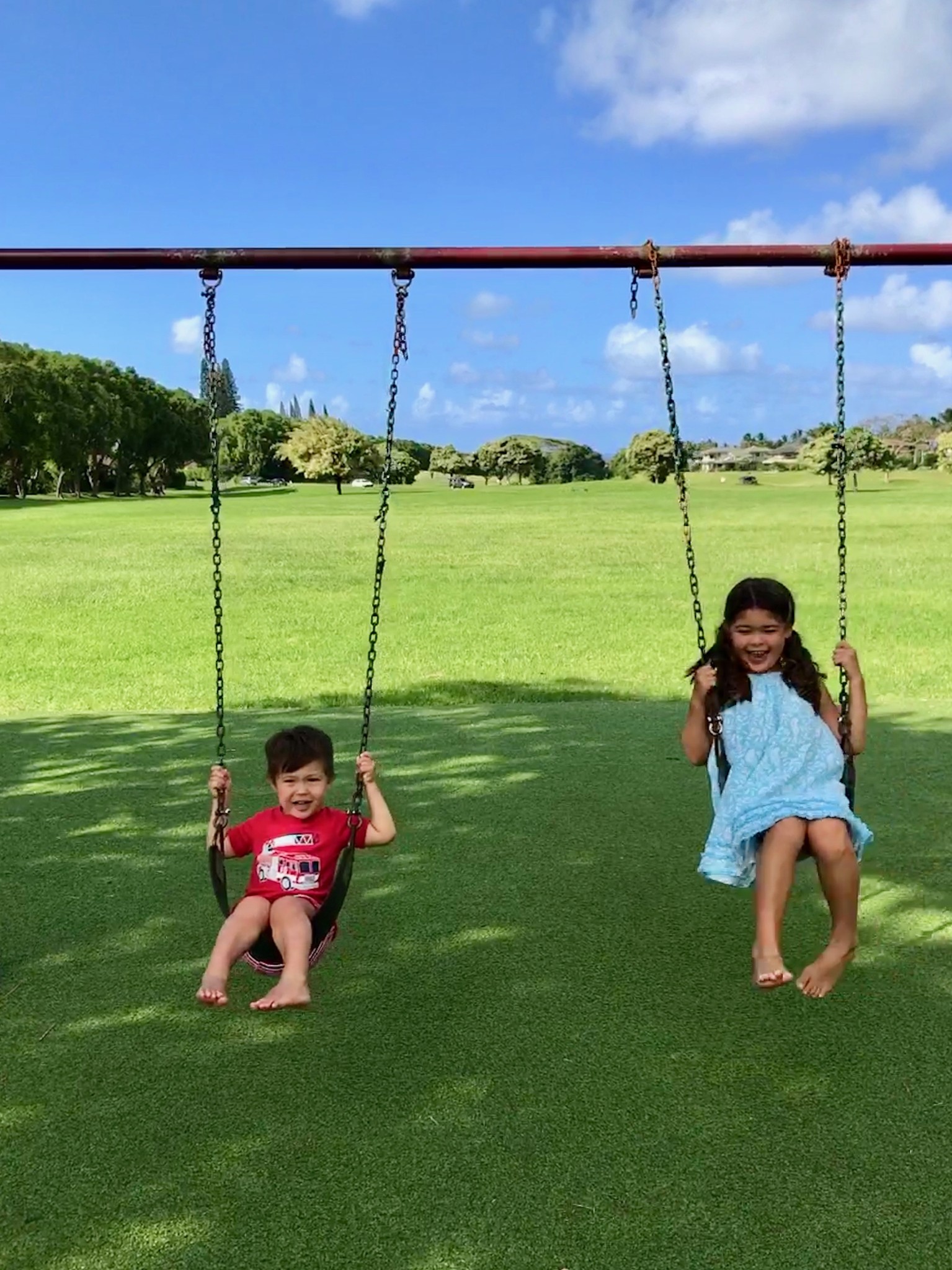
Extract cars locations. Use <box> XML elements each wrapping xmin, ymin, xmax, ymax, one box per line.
<box><xmin>351</xmin><ymin>478</ymin><xmax>374</xmax><ymax>488</ymax></box>
<box><xmin>270</xmin><ymin>478</ymin><xmax>287</xmax><ymax>488</ymax></box>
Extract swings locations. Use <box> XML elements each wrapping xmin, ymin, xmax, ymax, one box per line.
<box><xmin>198</xmin><ymin>272</ymin><xmax>419</xmax><ymax>974</ymax></box>
<box><xmin>628</xmin><ymin>235</ymin><xmax>856</xmax><ymax>814</ymax></box>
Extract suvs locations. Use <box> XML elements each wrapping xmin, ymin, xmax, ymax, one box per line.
<box><xmin>239</xmin><ymin>476</ymin><xmax>257</xmax><ymax>486</ymax></box>
<box><xmin>448</xmin><ymin>476</ymin><xmax>474</xmax><ymax>489</ymax></box>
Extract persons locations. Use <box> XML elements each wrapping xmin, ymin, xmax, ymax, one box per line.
<box><xmin>683</xmin><ymin>578</ymin><xmax>868</xmax><ymax>999</ymax></box>
<box><xmin>197</xmin><ymin>726</ymin><xmax>396</xmax><ymax>1010</ymax></box>
